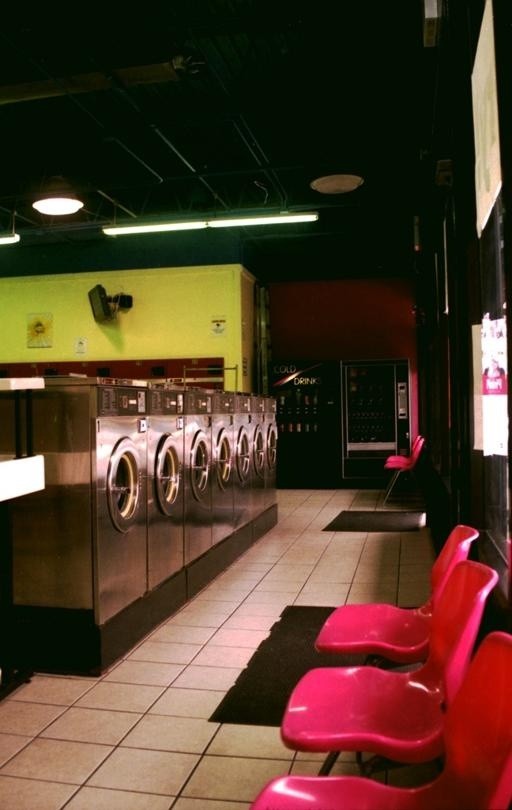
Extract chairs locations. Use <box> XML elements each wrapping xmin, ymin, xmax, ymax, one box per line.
<box><xmin>308</xmin><ymin>524</ymin><xmax>482</xmax><ymax>668</ymax></box>
<box><xmin>276</xmin><ymin>559</ymin><xmax>500</xmax><ymax>775</ymax></box>
<box><xmin>249</xmin><ymin>627</ymin><xmax>512</xmax><ymax>810</ymax></box>
<box><xmin>381</xmin><ymin>435</ymin><xmax>425</xmax><ymax>506</ymax></box>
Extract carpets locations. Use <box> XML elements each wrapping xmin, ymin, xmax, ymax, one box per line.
<box><xmin>208</xmin><ymin>606</ymin><xmax>421</xmax><ymax>726</ymax></box>
<box><xmin>323</xmin><ymin>512</ymin><xmax>426</xmax><ymax>531</ymax></box>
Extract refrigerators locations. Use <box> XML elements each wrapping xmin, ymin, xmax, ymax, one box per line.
<box><xmin>270</xmin><ymin>356</ymin><xmax>412</xmax><ymax>489</ymax></box>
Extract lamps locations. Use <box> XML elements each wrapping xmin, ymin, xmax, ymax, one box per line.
<box><xmin>310</xmin><ymin>173</ymin><xmax>364</xmax><ymax>195</ymax></box>
<box><xmin>31</xmin><ymin>170</ymin><xmax>83</xmax><ymax>221</ymax></box>
<box><xmin>0</xmin><ymin>205</ymin><xmax>21</xmax><ymax>245</ymax></box>
<box><xmin>102</xmin><ymin>199</ymin><xmax>320</xmax><ymax>235</ymax></box>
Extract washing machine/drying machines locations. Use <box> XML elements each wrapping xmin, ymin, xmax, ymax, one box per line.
<box><xmin>0</xmin><ymin>378</ymin><xmax>277</xmax><ymax>678</ymax></box>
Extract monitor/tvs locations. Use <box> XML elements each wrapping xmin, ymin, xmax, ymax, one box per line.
<box><xmin>87</xmin><ymin>285</ymin><xmax>112</xmax><ymax>325</ymax></box>
